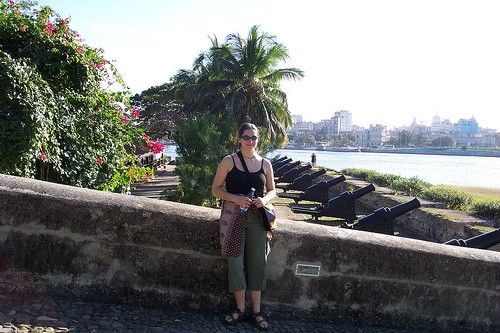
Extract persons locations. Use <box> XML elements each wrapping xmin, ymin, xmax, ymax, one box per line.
<box><xmin>212</xmin><ymin>122</ymin><xmax>277</xmax><ymax>329</ymax></box>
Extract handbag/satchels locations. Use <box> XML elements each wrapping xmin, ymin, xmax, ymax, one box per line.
<box><xmin>260</xmin><ymin>207</ymin><xmax>270</xmax><ymax>231</ymax></box>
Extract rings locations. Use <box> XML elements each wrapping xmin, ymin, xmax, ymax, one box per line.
<box><xmin>258</xmin><ymin>202</ymin><xmax>260</xmax><ymax>204</ymax></box>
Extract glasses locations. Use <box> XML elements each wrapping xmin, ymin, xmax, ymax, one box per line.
<box><xmin>240</xmin><ymin>135</ymin><xmax>258</xmax><ymax>141</ymax></box>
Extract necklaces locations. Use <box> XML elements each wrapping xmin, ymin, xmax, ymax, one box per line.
<box><xmin>243</xmin><ymin>154</ymin><xmax>257</xmax><ymax>162</ymax></box>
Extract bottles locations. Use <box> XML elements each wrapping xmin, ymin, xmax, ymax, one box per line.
<box><xmin>240</xmin><ymin>188</ymin><xmax>255</xmax><ymax>215</ymax></box>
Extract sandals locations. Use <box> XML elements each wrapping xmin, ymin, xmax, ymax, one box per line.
<box><xmin>223</xmin><ymin>307</ymin><xmax>247</xmax><ymax>325</ymax></box>
<box><xmin>250</xmin><ymin>311</ymin><xmax>269</xmax><ymax>330</ymax></box>
